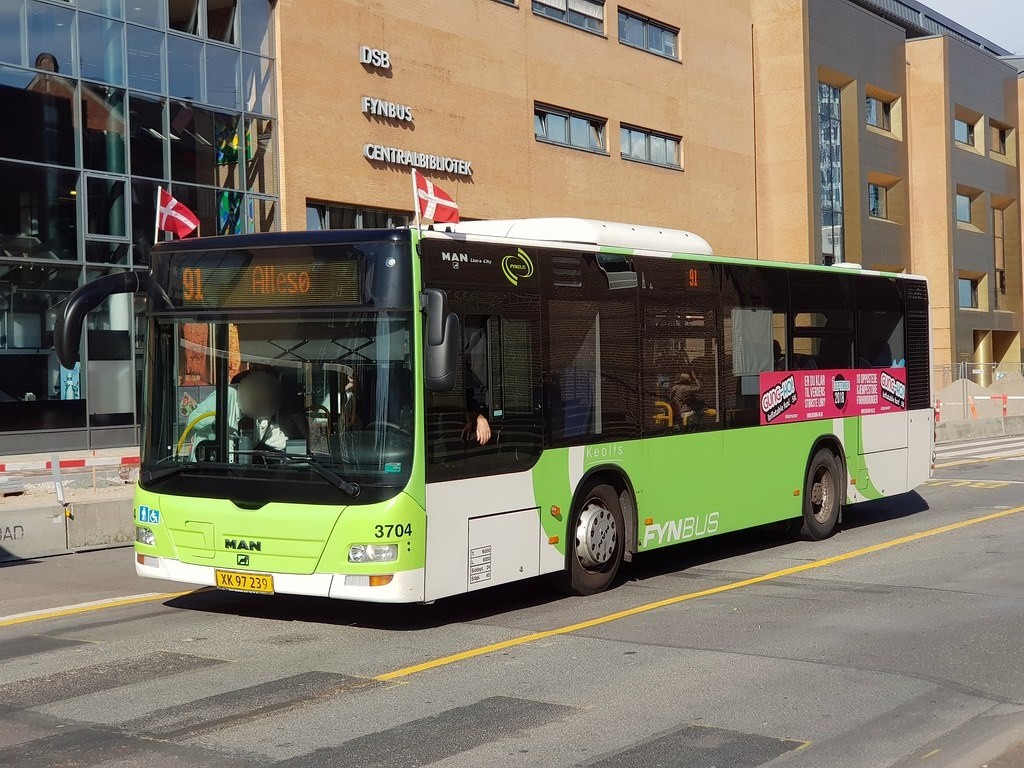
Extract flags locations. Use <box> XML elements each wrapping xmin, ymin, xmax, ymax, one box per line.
<box><xmin>156</xmin><ymin>186</ymin><xmax>200</xmax><ymax>238</ymax></box>
<box><xmin>412</xmin><ymin>169</ymin><xmax>460</xmax><ymax>225</ymax></box>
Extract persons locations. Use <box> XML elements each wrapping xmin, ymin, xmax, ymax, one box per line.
<box><xmin>253</xmin><ymin>410</ymin><xmax>292</xmax><ymax>451</ymax></box>
<box><xmin>669</xmin><ymin>367</ymin><xmax>706</xmax><ymax>432</ymax></box>
<box><xmin>431</xmin><ymin>377</ymin><xmax>491</xmax><ymax>445</ymax></box>
<box><xmin>772</xmin><ymin>339</ymin><xmax>788</xmax><ymax>372</ymax></box>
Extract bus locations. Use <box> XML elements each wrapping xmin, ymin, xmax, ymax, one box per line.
<box><xmin>55</xmin><ymin>216</ymin><xmax>938</xmax><ymax>607</ymax></box>
